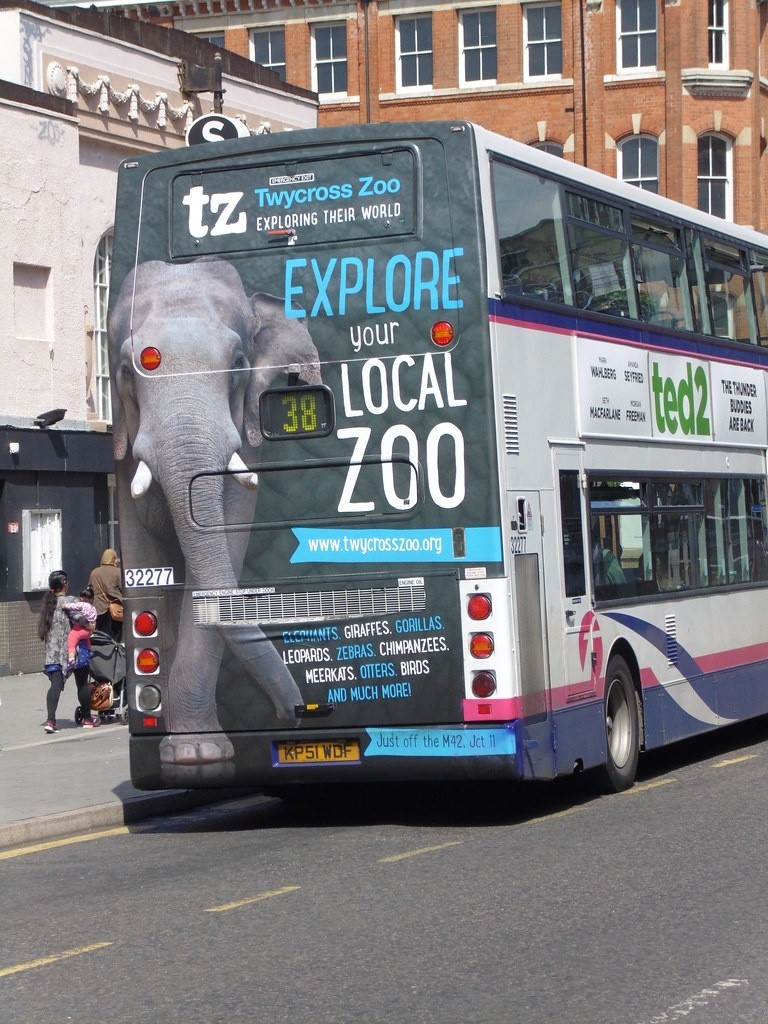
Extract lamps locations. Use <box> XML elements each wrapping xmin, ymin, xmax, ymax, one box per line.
<box><xmin>9</xmin><ymin>442</ymin><xmax>19</xmax><ymax>454</ymax></box>
<box><xmin>33</xmin><ymin>408</ymin><xmax>68</xmax><ymax>427</ymax></box>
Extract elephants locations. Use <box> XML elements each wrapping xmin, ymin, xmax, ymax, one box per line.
<box><xmin>110</xmin><ymin>251</ymin><xmax>322</xmax><ymax>762</ymax></box>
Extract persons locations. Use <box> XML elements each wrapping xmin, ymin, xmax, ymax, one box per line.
<box><xmin>37</xmin><ymin>570</ymin><xmax>101</xmax><ymax>733</ymax></box>
<box><xmin>590</xmin><ymin>533</ymin><xmax>628</xmax><ymax>586</ymax></box>
<box><xmin>89</xmin><ymin>549</ymin><xmax>124</xmax><ymax>642</ymax></box>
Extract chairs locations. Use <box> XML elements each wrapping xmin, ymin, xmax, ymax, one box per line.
<box><xmin>502</xmin><ymin>273</ymin><xmax>678</xmax><ymax>327</ymax></box>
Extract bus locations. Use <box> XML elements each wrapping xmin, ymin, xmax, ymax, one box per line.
<box><xmin>109</xmin><ymin>117</ymin><xmax>768</xmax><ymax>788</ymax></box>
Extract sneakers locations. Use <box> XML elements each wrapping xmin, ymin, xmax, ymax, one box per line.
<box><xmin>44</xmin><ymin>720</ymin><xmax>60</xmax><ymax>732</ymax></box>
<box><xmin>81</xmin><ymin>717</ymin><xmax>101</xmax><ymax>729</ymax></box>
<box><xmin>67</xmin><ymin>659</ymin><xmax>77</xmax><ymax>670</ymax></box>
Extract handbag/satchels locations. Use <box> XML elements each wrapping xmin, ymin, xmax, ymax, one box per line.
<box><xmin>88</xmin><ymin>680</ymin><xmax>114</xmax><ymax>711</ymax></box>
<box><xmin>108</xmin><ymin>602</ymin><xmax>123</xmax><ymax>622</ymax></box>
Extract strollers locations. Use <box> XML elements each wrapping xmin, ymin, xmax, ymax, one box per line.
<box><xmin>75</xmin><ymin>630</ymin><xmax>129</xmax><ymax>725</ymax></box>
